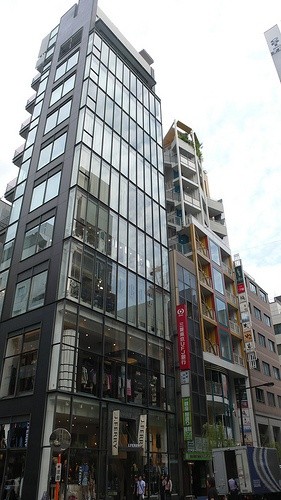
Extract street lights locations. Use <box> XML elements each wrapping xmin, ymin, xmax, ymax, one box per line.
<box><xmin>238</xmin><ymin>382</ymin><xmax>275</xmax><ymax>447</ymax></box>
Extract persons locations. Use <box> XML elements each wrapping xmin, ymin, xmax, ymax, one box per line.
<box><xmin>160</xmin><ymin>476</ymin><xmax>166</xmax><ymax>500</ymax></box>
<box><xmin>137</xmin><ymin>476</ymin><xmax>145</xmax><ymax>500</ymax></box>
<box><xmin>78</xmin><ymin>463</ymin><xmax>89</xmax><ymax>500</ymax></box>
<box><xmin>80</xmin><ymin>364</ymin><xmax>88</xmax><ymax>392</ymax></box>
<box><xmin>228</xmin><ymin>475</ymin><xmax>238</xmax><ymax>500</ymax></box>
<box><xmin>151</xmin><ymin>461</ymin><xmax>160</xmax><ymax>494</ymax></box>
<box><xmin>208</xmin><ymin>478</ymin><xmax>217</xmax><ymax>500</ymax></box>
<box><xmin>130</xmin><ymin>464</ymin><xmax>139</xmax><ymax>495</ymax></box>
<box><xmin>51</xmin><ymin>459</ymin><xmax>64</xmax><ymax>484</ymax></box>
<box><xmin>127</xmin><ymin>377</ymin><xmax>132</xmax><ymax>403</ymax></box>
<box><xmin>165</xmin><ymin>476</ymin><xmax>173</xmax><ymax>500</ymax></box>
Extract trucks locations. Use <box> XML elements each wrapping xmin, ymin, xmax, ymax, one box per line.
<box><xmin>211</xmin><ymin>446</ymin><xmax>281</xmax><ymax>500</ymax></box>
